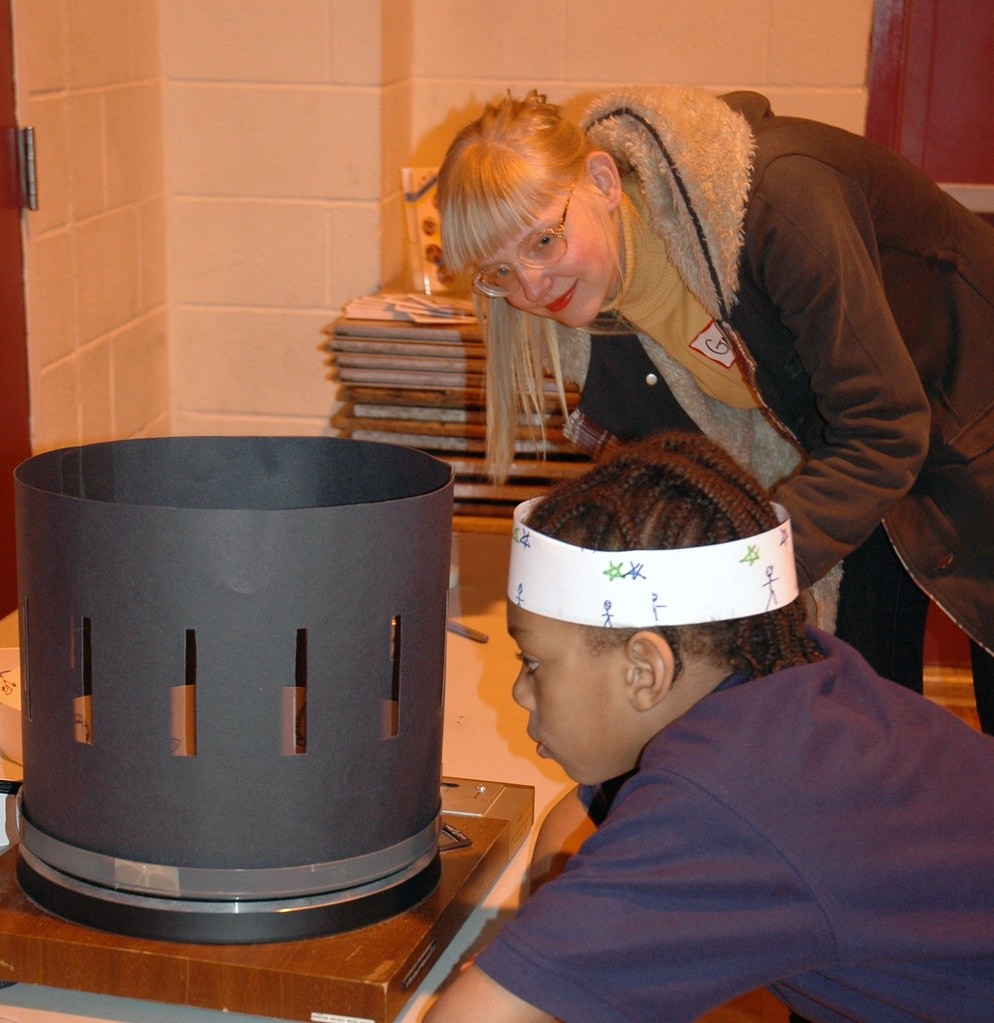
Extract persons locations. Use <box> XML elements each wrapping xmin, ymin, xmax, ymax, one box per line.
<box><xmin>438</xmin><ymin>87</ymin><xmax>994</xmax><ymax>735</ymax></box>
<box><xmin>421</xmin><ymin>434</ymin><xmax>994</xmax><ymax>1023</ymax></box>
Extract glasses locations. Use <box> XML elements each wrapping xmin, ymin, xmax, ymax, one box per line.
<box><xmin>472</xmin><ymin>180</ymin><xmax>574</xmax><ymax>299</ymax></box>
